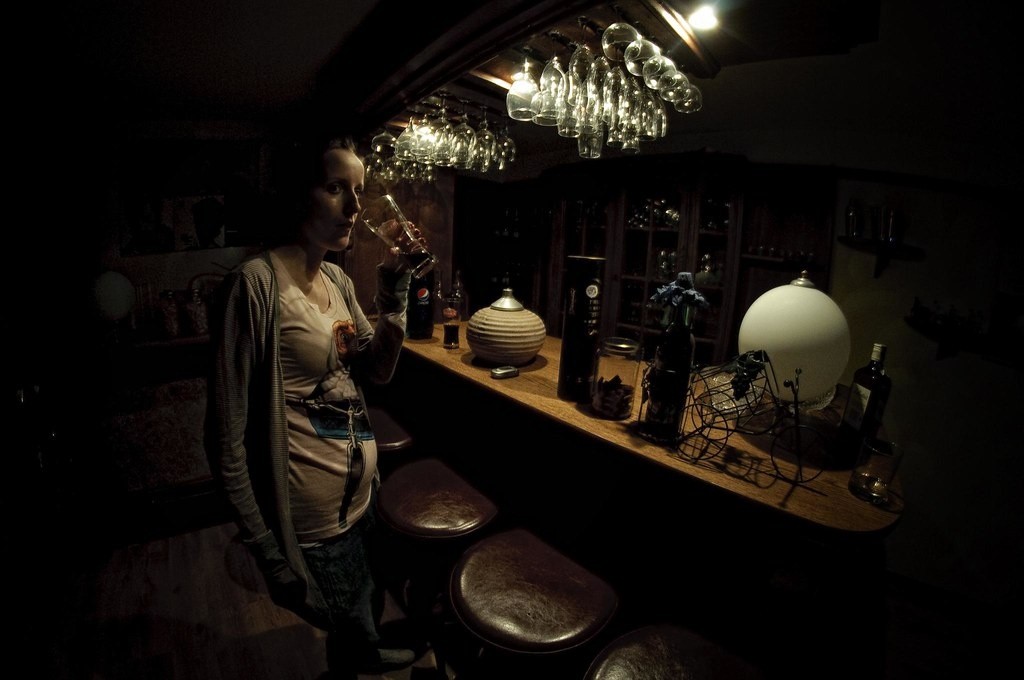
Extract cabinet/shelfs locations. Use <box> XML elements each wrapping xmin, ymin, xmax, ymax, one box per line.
<box><xmin>97</xmin><ymin>138</ymin><xmax>264</xmax><ymax>539</ymax></box>
<box><xmin>460</xmin><ymin>147</ymin><xmax>845</xmax><ymax>368</ymax></box>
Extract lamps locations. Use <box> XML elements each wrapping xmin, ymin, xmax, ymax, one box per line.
<box><xmin>738</xmin><ymin>270</ymin><xmax>850</xmax><ymax>436</ymax></box>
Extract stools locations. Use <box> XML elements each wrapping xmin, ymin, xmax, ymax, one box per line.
<box><xmin>583</xmin><ymin>622</ymin><xmax>763</xmax><ymax>680</ymax></box>
<box><xmin>375</xmin><ymin>457</ymin><xmax>501</xmax><ymax>627</ymax></box>
<box><xmin>450</xmin><ymin>526</ymin><xmax>621</xmax><ymax>657</ymax></box>
<box><xmin>366</xmin><ymin>405</ymin><xmax>415</xmax><ymax>455</ymax></box>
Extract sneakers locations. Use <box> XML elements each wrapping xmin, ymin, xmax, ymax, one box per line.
<box><xmin>376</xmin><ymin>647</ymin><xmax>416</xmax><ymax>665</ymax></box>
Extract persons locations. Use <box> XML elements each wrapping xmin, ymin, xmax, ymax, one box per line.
<box><xmin>201</xmin><ymin>138</ymin><xmax>428</xmax><ymax>680</ymax></box>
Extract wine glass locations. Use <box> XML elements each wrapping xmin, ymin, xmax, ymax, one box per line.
<box><xmin>363</xmin><ymin>86</ymin><xmax>517</xmax><ymax>186</ymax></box>
<box><xmin>500</xmin><ymin>0</ymin><xmax>703</xmax><ymax>160</ymax></box>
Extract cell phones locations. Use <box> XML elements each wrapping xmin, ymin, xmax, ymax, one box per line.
<box><xmin>491</xmin><ymin>366</ymin><xmax>519</xmax><ymax>379</ymax></box>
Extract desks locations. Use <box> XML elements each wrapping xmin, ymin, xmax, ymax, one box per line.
<box><xmin>368</xmin><ymin>319</ymin><xmax>905</xmax><ymax>533</ymax></box>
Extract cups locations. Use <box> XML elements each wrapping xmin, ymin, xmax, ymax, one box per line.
<box><xmin>843</xmin><ymin>198</ymin><xmax>866</xmax><ymax>238</ymax></box>
<box><xmin>359</xmin><ymin>192</ymin><xmax>439</xmax><ymax>280</ymax></box>
<box><xmin>881</xmin><ymin>205</ymin><xmax>906</xmax><ymax>241</ymax></box>
<box><xmin>441</xmin><ymin>297</ymin><xmax>462</xmax><ymax>350</ymax></box>
<box><xmin>847</xmin><ymin>435</ymin><xmax>903</xmax><ymax>506</ymax></box>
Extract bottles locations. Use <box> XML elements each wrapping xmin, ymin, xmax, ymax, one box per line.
<box><xmin>189</xmin><ymin>288</ymin><xmax>210</xmax><ymax>336</ymax></box>
<box><xmin>635</xmin><ymin>269</ymin><xmax>695</xmax><ymax>445</ymax></box>
<box><xmin>403</xmin><ymin>251</ymin><xmax>435</xmax><ymax>340</ymax></box>
<box><xmin>588</xmin><ymin>336</ymin><xmax>639</xmax><ymax>422</ymax></box>
<box><xmin>159</xmin><ymin>289</ymin><xmax>179</xmax><ymax>338</ymax></box>
<box><xmin>838</xmin><ymin>342</ymin><xmax>892</xmax><ymax>445</ymax></box>
